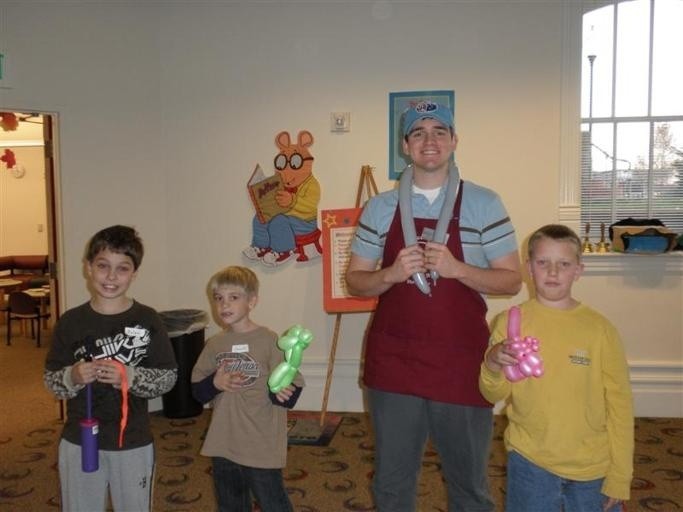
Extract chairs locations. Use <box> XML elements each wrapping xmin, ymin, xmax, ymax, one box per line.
<box><xmin>0</xmin><ymin>255</ymin><xmax>49</xmax><ymax>348</ymax></box>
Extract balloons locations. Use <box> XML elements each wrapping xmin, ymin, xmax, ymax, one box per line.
<box><xmin>399</xmin><ymin>161</ymin><xmax>460</xmax><ymax>296</ymax></box>
<box><xmin>267</xmin><ymin>324</ymin><xmax>314</xmax><ymax>394</ymax></box>
<box><xmin>500</xmin><ymin>306</ymin><xmax>545</xmax><ymax>383</ymax></box>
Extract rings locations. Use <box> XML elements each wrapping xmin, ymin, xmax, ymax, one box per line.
<box><xmin>427</xmin><ymin>256</ymin><xmax>431</xmax><ymax>264</ymax></box>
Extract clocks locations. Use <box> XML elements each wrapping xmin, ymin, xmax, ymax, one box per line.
<box><xmin>12</xmin><ymin>163</ymin><xmax>26</xmax><ymax>178</ymax></box>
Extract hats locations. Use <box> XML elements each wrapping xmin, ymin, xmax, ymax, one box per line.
<box><xmin>403</xmin><ymin>101</ymin><xmax>453</xmax><ymax>136</ymax></box>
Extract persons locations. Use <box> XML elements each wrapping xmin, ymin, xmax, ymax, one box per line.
<box><xmin>478</xmin><ymin>224</ymin><xmax>635</xmax><ymax>511</ymax></box>
<box><xmin>43</xmin><ymin>225</ymin><xmax>178</xmax><ymax>511</ymax></box>
<box><xmin>188</xmin><ymin>265</ymin><xmax>304</xmax><ymax>511</ymax></box>
<box><xmin>343</xmin><ymin>101</ymin><xmax>521</xmax><ymax>511</ymax></box>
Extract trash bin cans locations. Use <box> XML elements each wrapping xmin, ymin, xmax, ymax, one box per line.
<box><xmin>160</xmin><ymin>309</ymin><xmax>206</xmax><ymax>419</ymax></box>
